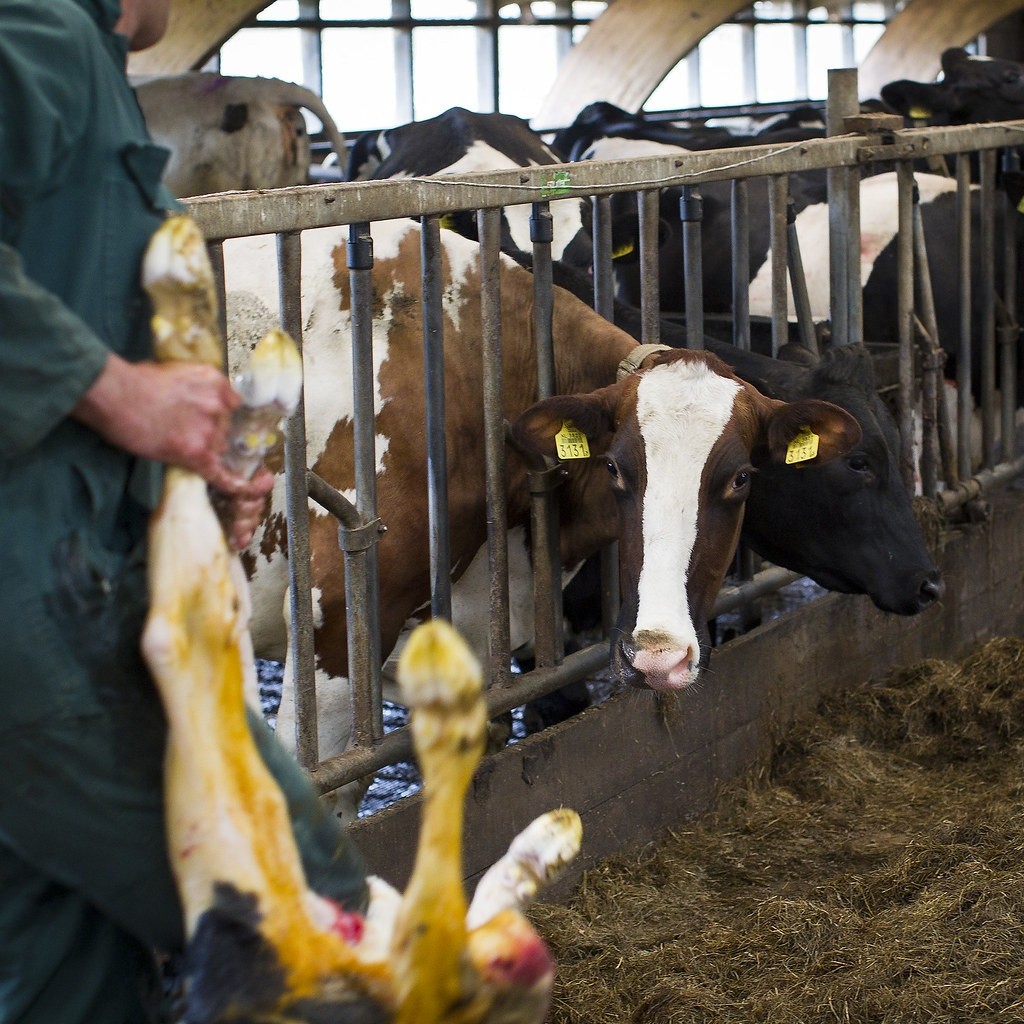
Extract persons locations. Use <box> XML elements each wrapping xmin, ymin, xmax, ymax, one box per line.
<box><xmin>0</xmin><ymin>0</ymin><xmax>373</xmax><ymax>1024</ymax></box>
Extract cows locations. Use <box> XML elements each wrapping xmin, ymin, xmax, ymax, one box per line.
<box><xmin>118</xmin><ymin>48</ymin><xmax>1024</xmax><ymax>820</ymax></box>
